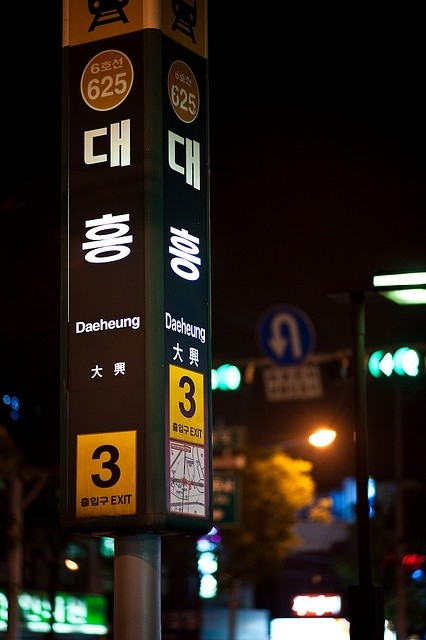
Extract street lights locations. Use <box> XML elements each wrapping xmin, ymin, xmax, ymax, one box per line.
<box><xmin>350</xmin><ymin>260</ymin><xmax>425</xmax><ymax>640</ymax></box>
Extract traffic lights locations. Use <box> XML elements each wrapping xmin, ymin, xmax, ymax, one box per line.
<box><xmin>365</xmin><ymin>346</ymin><xmax>420</xmax><ymax>381</ymax></box>
<box><xmin>210</xmin><ymin>363</ymin><xmax>247</xmax><ymax>392</ymax></box>
